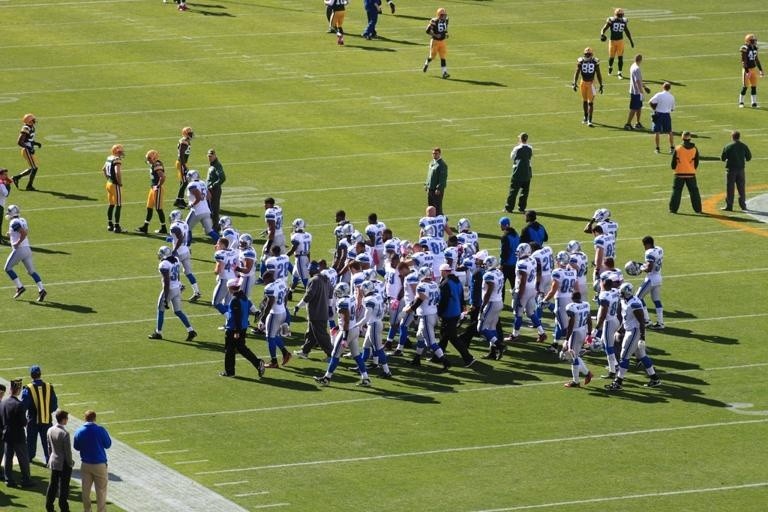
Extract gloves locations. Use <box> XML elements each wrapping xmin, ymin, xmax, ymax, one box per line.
<box><xmin>599</xmin><ymin>86</ymin><xmax>603</xmax><ymax>94</ymax></box>
<box><xmin>746</xmin><ymin>71</ymin><xmax>752</xmax><ymax>79</ymax></box>
<box><xmin>759</xmin><ymin>71</ymin><xmax>764</xmax><ymax>78</ymax></box>
<box><xmin>27</xmin><ymin>146</ymin><xmax>34</xmax><ymax>154</ymax></box>
<box><xmin>639</xmin><ymin>93</ymin><xmax>645</xmax><ymax>101</ymax></box>
<box><xmin>572</xmin><ymin>83</ymin><xmax>578</xmax><ymax>92</ymax></box>
<box><xmin>34</xmin><ymin>142</ymin><xmax>41</xmax><ymax>148</ymax></box>
<box><xmin>644</xmin><ymin>87</ymin><xmax>650</xmax><ymax>94</ymax></box>
<box><xmin>601</xmin><ymin>35</ymin><xmax>607</xmax><ymax>41</ymax></box>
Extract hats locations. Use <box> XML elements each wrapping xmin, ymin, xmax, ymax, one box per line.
<box><xmin>32</xmin><ymin>366</ymin><xmax>40</xmax><ymax>377</ymax></box>
<box><xmin>208</xmin><ymin>149</ymin><xmax>215</xmax><ymax>155</ymax></box>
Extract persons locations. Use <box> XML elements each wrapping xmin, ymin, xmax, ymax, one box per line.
<box><xmin>102</xmin><ymin>128</ymin><xmax>226</xmax><ymax>245</ymax></box>
<box><xmin>323</xmin><ymin>0</ymin><xmax>396</xmax><ymax>45</ymax></box>
<box><xmin>10</xmin><ymin>113</ymin><xmax>41</xmax><ymax>192</ymax></box>
<box><xmin>424</xmin><ymin>148</ymin><xmax>448</xmax><ymax>215</ymax></box>
<box><xmin>572</xmin><ymin>47</ymin><xmax>605</xmax><ymax>128</ymax></box>
<box><xmin>669</xmin><ymin>131</ymin><xmax>702</xmax><ymax>214</ymax></box>
<box><xmin>422</xmin><ymin>8</ymin><xmax>451</xmax><ymax>79</ymax></box>
<box><xmin>500</xmin><ymin>133</ymin><xmax>533</xmax><ymax>212</ymax></box>
<box><xmin>1</xmin><ymin>366</ymin><xmax>111</xmax><ymax>512</ymax></box>
<box><xmin>0</xmin><ymin>204</ymin><xmax>47</xmax><ymax>302</ymax></box>
<box><xmin>649</xmin><ymin>81</ymin><xmax>676</xmax><ymax>155</ymax></box>
<box><xmin>0</xmin><ymin>169</ymin><xmax>13</xmax><ymax>244</ymax></box>
<box><xmin>174</xmin><ymin>0</ymin><xmax>188</xmax><ymax>12</ymax></box>
<box><xmin>624</xmin><ymin>55</ymin><xmax>650</xmax><ymax>131</ymax></box>
<box><xmin>600</xmin><ymin>8</ymin><xmax>634</xmax><ymax>80</ymax></box>
<box><xmin>721</xmin><ymin>132</ymin><xmax>752</xmax><ymax>212</ymax></box>
<box><xmin>148</xmin><ymin>170</ymin><xmax>665</xmax><ymax>391</ymax></box>
<box><xmin>738</xmin><ymin>34</ymin><xmax>765</xmax><ymax>109</ymax></box>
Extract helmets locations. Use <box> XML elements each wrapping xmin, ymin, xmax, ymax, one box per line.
<box><xmin>590</xmin><ymin>336</ymin><xmax>604</xmax><ymax>351</ymax></box>
<box><xmin>681</xmin><ymin>131</ymin><xmax>691</xmax><ymax>140</ymax></box>
<box><xmin>158</xmin><ymin>206</ymin><xmax>582</xmax><ymax>299</ymax></box>
<box><xmin>744</xmin><ymin>34</ymin><xmax>757</xmax><ymax>47</ymax></box>
<box><xmin>620</xmin><ymin>282</ymin><xmax>635</xmax><ymax>299</ymax></box>
<box><xmin>625</xmin><ymin>261</ymin><xmax>642</xmax><ymax>276</ymax></box>
<box><xmin>615</xmin><ymin>8</ymin><xmax>625</xmax><ymax>19</ymax></box>
<box><xmin>436</xmin><ymin>7</ymin><xmax>447</xmax><ymax>21</ymax></box>
<box><xmin>595</xmin><ymin>209</ymin><xmax>611</xmax><ymax>221</ymax></box>
<box><xmin>23</xmin><ymin>114</ymin><xmax>38</xmax><ymax>129</ymax></box>
<box><xmin>6</xmin><ymin>204</ymin><xmax>19</xmax><ymax>218</ymax></box>
<box><xmin>111</xmin><ymin>126</ymin><xmax>200</xmax><ymax>182</ymax></box>
<box><xmin>582</xmin><ymin>47</ymin><xmax>595</xmax><ymax>60</ymax></box>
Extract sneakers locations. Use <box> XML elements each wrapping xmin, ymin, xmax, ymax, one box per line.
<box><xmin>647</xmin><ymin>320</ymin><xmax>652</xmax><ymax>330</ymax></box>
<box><xmin>1</xmin><ymin>239</ymin><xmax>8</xmax><ymax>244</ymax></box>
<box><xmin>36</xmin><ymin>289</ymin><xmax>47</xmax><ymax>301</ymax></box>
<box><xmin>544</xmin><ymin>344</ymin><xmax>560</xmax><ymax>354</ymax></box>
<box><xmin>669</xmin><ymin>207</ymin><xmax>746</xmax><ymax>214</ymax></box>
<box><xmin>584</xmin><ymin>370</ymin><xmax>593</xmax><ymax>385</ymax></box>
<box><xmin>649</xmin><ymin>322</ymin><xmax>665</xmax><ymax>329</ymax></box>
<box><xmin>26</xmin><ymin>185</ymin><xmax>35</xmax><ymax>191</ymax></box>
<box><xmin>106</xmin><ymin>198</ymin><xmax>188</xmax><ymax>234</ymax></box>
<box><xmin>423</xmin><ymin>65</ymin><xmax>428</xmax><ymax>73</ymax></box>
<box><xmin>185</xmin><ymin>331</ymin><xmax>197</xmax><ymax>342</ymax></box>
<box><xmin>564</xmin><ymin>381</ymin><xmax>580</xmax><ymax>388</ymax></box>
<box><xmin>552</xmin><ymin>306</ymin><xmax>556</xmax><ymax>320</ymax></box>
<box><xmin>189</xmin><ymin>292</ymin><xmax>202</xmax><ymax>303</ymax></box>
<box><xmin>11</xmin><ymin>176</ymin><xmax>18</xmax><ymax>188</ymax></box>
<box><xmin>443</xmin><ymin>72</ymin><xmax>450</xmax><ymax>79</ymax></box>
<box><xmin>739</xmin><ymin>104</ymin><xmax>744</xmax><ymax>108</ymax></box>
<box><xmin>326</xmin><ymin>26</ymin><xmax>380</xmax><ymax>45</ymax></box>
<box><xmin>217</xmin><ymin>300</ymin><xmax>547</xmax><ymax>387</ymax></box>
<box><xmin>179</xmin><ymin>5</ymin><xmax>186</xmax><ymax>11</ymax></box>
<box><xmin>599</xmin><ymin>357</ymin><xmax>662</xmax><ymax>391</ymax></box>
<box><xmin>149</xmin><ymin>330</ymin><xmax>162</xmax><ymax>341</ymax></box>
<box><xmin>13</xmin><ymin>287</ymin><xmax>25</xmax><ymax>298</ymax></box>
<box><xmin>582</xmin><ymin>67</ymin><xmax>675</xmax><ymax>154</ymax></box>
<box><xmin>752</xmin><ymin>105</ymin><xmax>760</xmax><ymax>109</ymax></box>
